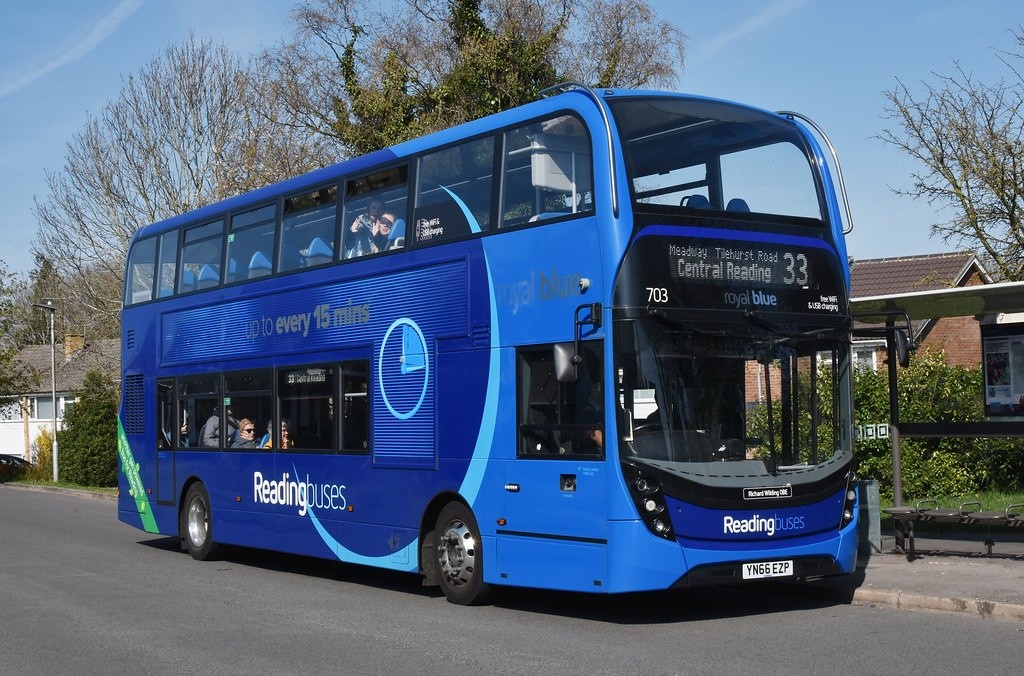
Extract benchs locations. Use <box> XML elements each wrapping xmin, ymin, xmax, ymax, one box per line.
<box><xmin>882</xmin><ymin>500</ymin><xmax>1024</xmax><ymax>563</ymax></box>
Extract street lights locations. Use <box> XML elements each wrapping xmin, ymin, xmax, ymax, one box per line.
<box><xmin>33</xmin><ymin>303</ymin><xmax>58</xmax><ymax>483</ymax></box>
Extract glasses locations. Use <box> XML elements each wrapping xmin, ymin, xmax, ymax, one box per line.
<box><xmin>243</xmin><ymin>428</ymin><xmax>256</xmax><ymax>433</ymax></box>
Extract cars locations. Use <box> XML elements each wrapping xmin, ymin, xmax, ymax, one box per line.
<box><xmin>0</xmin><ymin>453</ymin><xmax>35</xmax><ymax>483</ymax></box>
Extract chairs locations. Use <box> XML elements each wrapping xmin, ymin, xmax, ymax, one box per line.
<box><xmin>681</xmin><ymin>195</ymin><xmax>712</xmax><ymax>208</ymax></box>
<box><xmin>726</xmin><ymin>197</ymin><xmax>750</xmax><ymax>212</ymax></box>
<box><xmin>184</xmin><ymin>429</ymin><xmax>270</xmax><ymax>448</ymax></box>
<box><xmin>158</xmin><ymin>220</ymin><xmax>407</xmax><ymax>298</ymax></box>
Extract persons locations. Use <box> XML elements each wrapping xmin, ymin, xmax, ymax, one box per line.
<box><xmin>163</xmin><ymin>397</ymin><xmax>296</xmax><ymax>454</ymax></box>
<box><xmin>992</xmin><ymin>367</ymin><xmax>1008</xmax><ymax>385</ymax></box>
<box><xmin>344</xmin><ymin>196</ymin><xmax>398</xmax><ymax>259</ymax></box>
<box><xmin>572</xmin><ymin>379</ymin><xmax>623</xmax><ymax>455</ymax></box>
<box><xmin>645</xmin><ymin>386</ymin><xmax>687</xmax><ymax>450</ymax></box>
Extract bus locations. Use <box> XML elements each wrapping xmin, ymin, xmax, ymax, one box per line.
<box><xmin>117</xmin><ymin>80</ymin><xmax>915</xmax><ymax>607</ymax></box>
